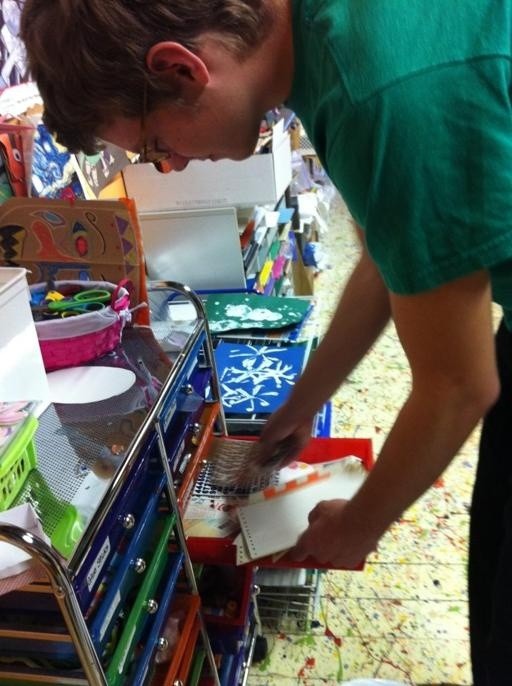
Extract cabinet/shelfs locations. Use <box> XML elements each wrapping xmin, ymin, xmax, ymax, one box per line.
<box><xmin>0</xmin><ymin>279</ymin><xmax>268</xmax><ymax>686</ymax></box>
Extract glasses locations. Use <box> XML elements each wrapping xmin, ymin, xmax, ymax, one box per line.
<box><xmin>132</xmin><ymin>79</ymin><xmax>171</xmax><ymax>164</ymax></box>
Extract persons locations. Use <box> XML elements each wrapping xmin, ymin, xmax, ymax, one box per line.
<box><xmin>17</xmin><ymin>1</ymin><xmax>511</xmax><ymax>680</ymax></box>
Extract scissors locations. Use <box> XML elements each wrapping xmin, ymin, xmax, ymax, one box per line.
<box><xmin>32</xmin><ymin>289</ymin><xmax>111</xmax><ymax>316</ymax></box>
